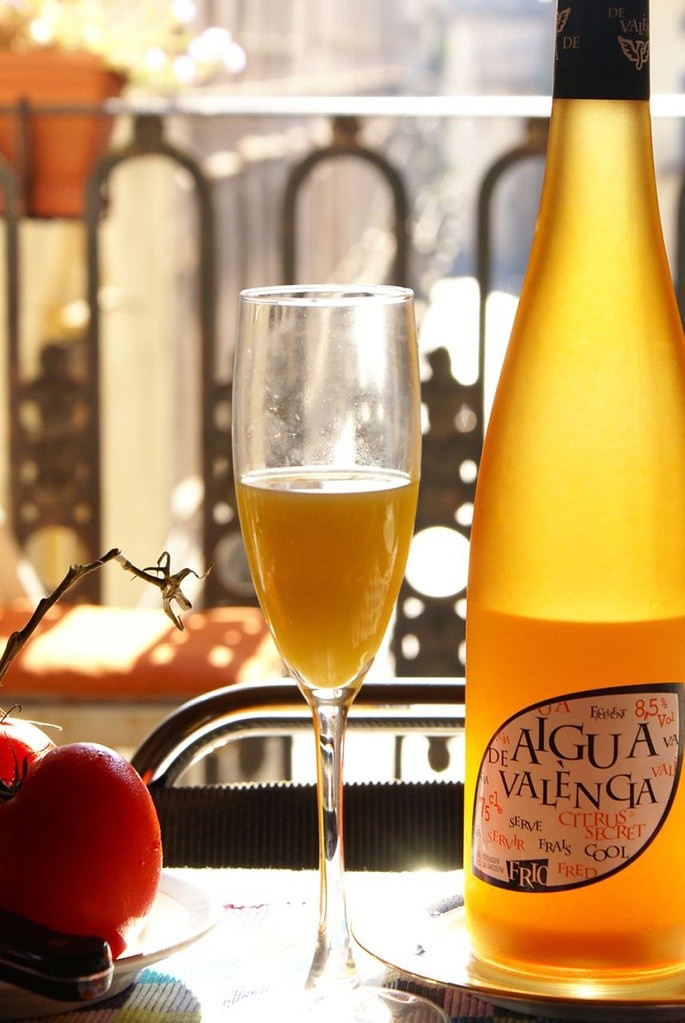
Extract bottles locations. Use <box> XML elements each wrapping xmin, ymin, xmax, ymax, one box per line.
<box><xmin>457</xmin><ymin>0</ymin><xmax>685</xmax><ymax>995</ymax></box>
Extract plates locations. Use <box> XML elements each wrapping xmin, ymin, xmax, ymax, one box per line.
<box><xmin>0</xmin><ymin>874</ymin><xmax>221</xmax><ymax>1012</ymax></box>
<box><xmin>344</xmin><ymin>863</ymin><xmax>684</xmax><ymax>1020</ymax></box>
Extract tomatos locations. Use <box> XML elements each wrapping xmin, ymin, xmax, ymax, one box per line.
<box><xmin>0</xmin><ymin>705</ymin><xmax>164</xmax><ymax>957</ymax></box>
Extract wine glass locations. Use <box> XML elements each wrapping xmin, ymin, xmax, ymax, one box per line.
<box><xmin>222</xmin><ymin>277</ymin><xmax>448</xmax><ymax>1023</ymax></box>
